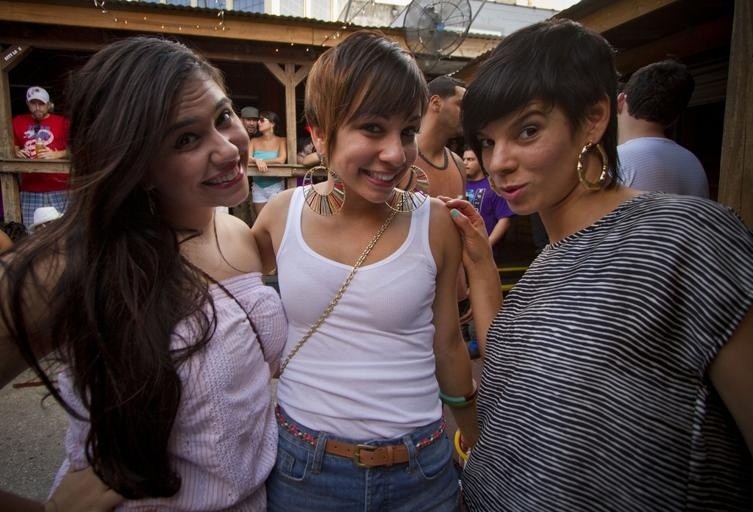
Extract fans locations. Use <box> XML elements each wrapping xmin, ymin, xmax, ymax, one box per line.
<box><xmin>401</xmin><ymin>1</ymin><xmax>472</xmax><ymax>61</ymax></box>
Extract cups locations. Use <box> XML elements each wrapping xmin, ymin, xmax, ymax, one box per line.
<box><xmin>34</xmin><ymin>137</ymin><xmax>45</xmax><ymax>159</ymax></box>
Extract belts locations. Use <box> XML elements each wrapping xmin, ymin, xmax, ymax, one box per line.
<box><xmin>321</xmin><ymin>439</ymin><xmax>408</xmax><ymax>467</ymax></box>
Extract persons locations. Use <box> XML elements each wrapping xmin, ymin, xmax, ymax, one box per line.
<box><xmin>297</xmin><ymin>136</ymin><xmax>319</xmax><ymax>187</ymax></box>
<box><xmin>230</xmin><ymin>104</ymin><xmax>258</xmax><ymax>229</ymax></box>
<box><xmin>0</xmin><ymin>32</ymin><xmax>288</xmax><ymax>511</ymax></box>
<box><xmin>610</xmin><ymin>60</ymin><xmax>711</xmax><ymax>200</ymax></box>
<box><xmin>396</xmin><ymin>74</ymin><xmax>472</xmax><ymax>327</ymax></box>
<box><xmin>11</xmin><ymin>83</ymin><xmax>78</xmax><ymax>236</ymax></box>
<box><xmin>435</xmin><ymin>17</ymin><xmax>753</xmax><ymax>512</ymax></box>
<box><xmin>26</xmin><ymin>204</ymin><xmax>65</xmax><ymax>239</ymax></box>
<box><xmin>248</xmin><ymin>110</ymin><xmax>288</xmax><ymax>277</ymax></box>
<box><xmin>247</xmin><ymin>29</ymin><xmax>484</xmax><ymax>512</ymax></box>
<box><xmin>460</xmin><ymin>146</ymin><xmax>513</xmax><ymax>361</ymax></box>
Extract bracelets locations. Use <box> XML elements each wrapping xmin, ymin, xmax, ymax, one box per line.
<box><xmin>437</xmin><ymin>378</ymin><xmax>479</xmax><ymax>408</ymax></box>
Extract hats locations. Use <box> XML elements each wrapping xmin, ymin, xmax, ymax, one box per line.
<box><xmin>241</xmin><ymin>107</ymin><xmax>259</xmax><ymax>118</ymax></box>
<box><xmin>27</xmin><ymin>87</ymin><xmax>49</xmax><ymax>104</ymax></box>
<box><xmin>28</xmin><ymin>207</ymin><xmax>63</xmax><ymax>231</ymax></box>
